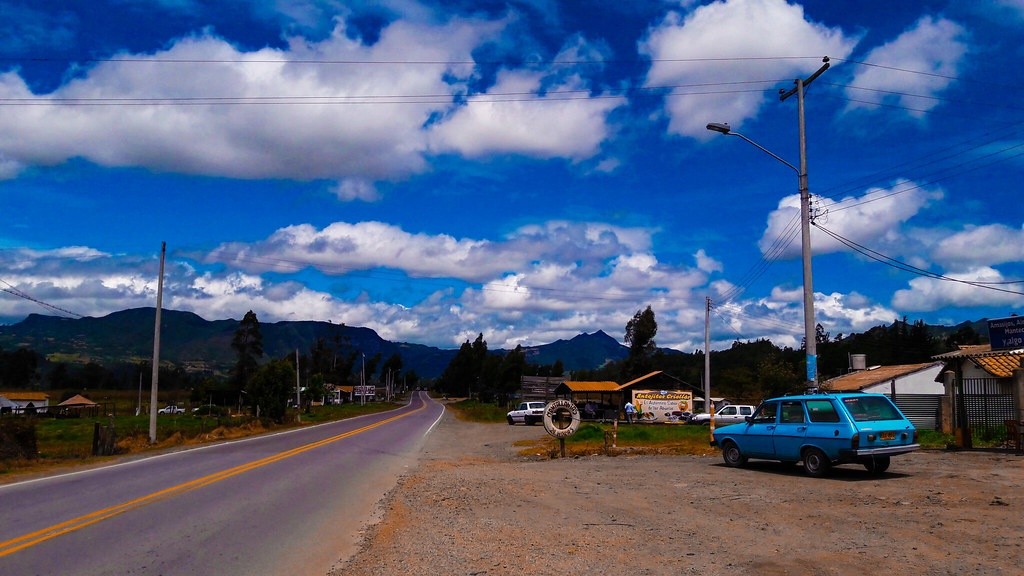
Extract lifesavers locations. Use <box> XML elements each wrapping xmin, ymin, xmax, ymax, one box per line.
<box><xmin>542</xmin><ymin>399</ymin><xmax>581</xmax><ymax>438</ymax></box>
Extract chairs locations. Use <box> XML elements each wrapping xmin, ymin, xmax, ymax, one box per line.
<box><xmin>1005</xmin><ymin>419</ymin><xmax>1024</xmax><ymax>456</ymax></box>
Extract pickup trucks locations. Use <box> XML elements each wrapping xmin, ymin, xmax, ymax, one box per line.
<box><xmin>157</xmin><ymin>405</ymin><xmax>186</xmax><ymax>414</ymax></box>
<box><xmin>691</xmin><ymin>404</ymin><xmax>781</xmax><ymax>430</ymax></box>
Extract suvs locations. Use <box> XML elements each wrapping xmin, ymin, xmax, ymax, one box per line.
<box><xmin>192</xmin><ymin>403</ymin><xmax>218</xmax><ymax>416</ymax></box>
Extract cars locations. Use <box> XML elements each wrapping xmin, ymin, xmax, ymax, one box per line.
<box><xmin>506</xmin><ymin>401</ymin><xmax>557</xmax><ymax>426</ymax></box>
<box><xmin>710</xmin><ymin>388</ymin><xmax>921</xmax><ymax>477</ymax></box>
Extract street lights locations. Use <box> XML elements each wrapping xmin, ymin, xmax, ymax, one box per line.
<box><xmin>707</xmin><ymin>54</ymin><xmax>839</xmax><ymax>393</ymax></box>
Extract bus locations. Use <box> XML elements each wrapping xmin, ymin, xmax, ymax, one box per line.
<box><xmin>328</xmin><ymin>388</ymin><xmax>349</xmax><ymax>406</ymax></box>
<box><xmin>309</xmin><ymin>392</ymin><xmax>327</xmax><ymax>406</ymax></box>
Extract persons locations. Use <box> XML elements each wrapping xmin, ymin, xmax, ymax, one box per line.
<box><xmin>583</xmin><ymin>399</ymin><xmax>612</xmax><ymax>420</ymax></box>
<box><xmin>624</xmin><ymin>400</ymin><xmax>636</xmax><ymax>424</ymax></box>
